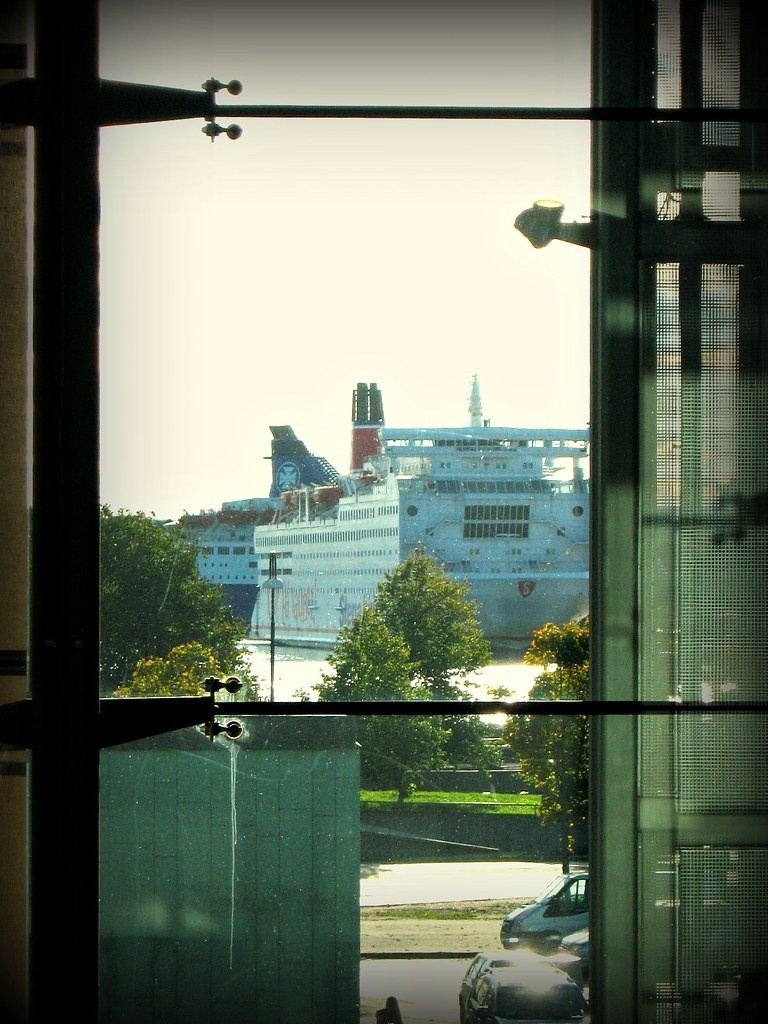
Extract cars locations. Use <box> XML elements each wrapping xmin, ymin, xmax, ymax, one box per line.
<box><xmin>458</xmin><ymin>949</ymin><xmax>591</xmax><ymax>1024</ymax></box>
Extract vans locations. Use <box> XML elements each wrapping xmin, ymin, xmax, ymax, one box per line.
<box><xmin>558</xmin><ymin>897</ymin><xmax>726</xmax><ymax>968</ymax></box>
<box><xmin>497</xmin><ymin>870</ymin><xmax>676</xmax><ymax>949</ymax></box>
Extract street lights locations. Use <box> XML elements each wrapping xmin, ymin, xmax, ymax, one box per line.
<box><xmin>262</xmin><ymin>552</ymin><xmax>286</xmax><ymax>702</ymax></box>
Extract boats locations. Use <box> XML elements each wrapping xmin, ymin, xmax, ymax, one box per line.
<box><xmin>312</xmin><ymin>485</ymin><xmax>342</xmax><ymax>506</ymax></box>
<box><xmin>278</xmin><ymin>490</ymin><xmax>293</xmax><ymax>505</ymax></box>
<box><xmin>292</xmin><ymin>486</ymin><xmax>314</xmax><ymax>504</ymax></box>
<box><xmin>236</xmin><ymin>638</ymin><xmax>278</xmax><ymax>648</ymax></box>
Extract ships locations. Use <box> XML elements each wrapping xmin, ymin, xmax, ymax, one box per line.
<box><xmin>250</xmin><ymin>373</ymin><xmax>592</xmax><ymax>650</ymax></box>
